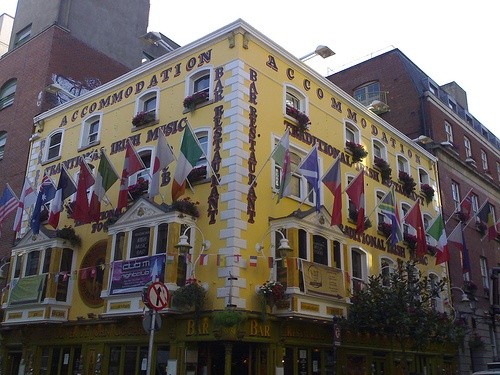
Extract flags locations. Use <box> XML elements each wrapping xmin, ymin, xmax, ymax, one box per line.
<box><xmin>117</xmin><ymin>141</ymin><xmax>146</xmax><ymax>214</ymax></box>
<box><xmin>272</xmin><ymin>132</ymin><xmax>291</xmax><ymax>204</ymax></box>
<box><xmin>28</xmin><ymin>173</ymin><xmax>57</xmax><ymax>233</ymax></box>
<box><xmin>381</xmin><ymin>186</ymin><xmax>403</xmax><ymax>247</ymax></box>
<box><xmin>301</xmin><ymin>147</ymin><xmax>322</xmax><ymax>213</ymax></box>
<box><xmin>449</xmin><ymin>224</ymin><xmax>472</xmax><ymax>273</ymax></box>
<box><xmin>324</xmin><ymin>157</ymin><xmax>344</xmax><ymax>227</ymax></box>
<box><xmin>346</xmin><ymin>170</ymin><xmax>366</xmax><ymax>235</ymax></box>
<box><xmin>172</xmin><ymin>124</ymin><xmax>204</xmax><ymax>200</ymax></box>
<box><xmin>167</xmin><ymin>252</ymin><xmax>301</xmax><ymax>269</ymax></box>
<box><xmin>88</xmin><ymin>153</ymin><xmax>119</xmax><ymax>223</ymax></box>
<box><xmin>54</xmin><ymin>264</ymin><xmax>105</xmax><ymax>283</ymax></box>
<box><xmin>427</xmin><ymin>213</ymin><xmax>451</xmax><ymax>265</ymax></box>
<box><xmin>48</xmin><ymin>166</ymin><xmax>76</xmax><ymax>229</ymax></box>
<box><xmin>406</xmin><ymin>201</ymin><xmax>428</xmax><ymax>257</ymax></box>
<box><xmin>72</xmin><ymin>160</ymin><xmax>95</xmax><ymax>223</ymax></box>
<box><xmin>149</xmin><ymin>126</ymin><xmax>173</xmax><ymax>202</ymax></box>
<box><xmin>479</xmin><ymin>202</ymin><xmax>498</xmax><ymax>241</ymax></box>
<box><xmin>463</xmin><ymin>191</ymin><xmax>473</xmax><ymax>217</ymax></box>
<box><xmin>0</xmin><ymin>185</ymin><xmax>19</xmax><ymax>225</ymax></box>
<box><xmin>13</xmin><ymin>178</ymin><xmax>36</xmax><ymax>230</ymax></box>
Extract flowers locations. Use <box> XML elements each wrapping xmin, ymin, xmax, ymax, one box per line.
<box><xmin>403</xmin><ymin>233</ymin><xmax>416</xmax><ymax>245</ymax></box>
<box><xmin>398</xmin><ymin>170</ymin><xmax>416</xmax><ymax>190</ymax></box>
<box><xmin>376</xmin><ymin>222</ymin><xmax>393</xmax><ymax>236</ymax></box>
<box><xmin>103</xmin><ymin>166</ymin><xmax>208</xmax><ymax>232</ymax></box>
<box><xmin>346</xmin><ymin>140</ymin><xmax>368</xmax><ymax>163</ymax></box>
<box><xmin>286</xmin><ymin>106</ymin><xmax>311</xmax><ymax>132</ymax></box>
<box><xmin>132</xmin><ymin>91</ymin><xmax>208</xmax><ymax>127</ymax></box>
<box><xmin>255</xmin><ymin>279</ymin><xmax>287</xmax><ymax>311</ymax></box>
<box><xmin>421</xmin><ymin>184</ymin><xmax>489</xmax><ymax>233</ymax></box>
<box><xmin>374</xmin><ymin>155</ymin><xmax>392</xmax><ymax>181</ymax></box>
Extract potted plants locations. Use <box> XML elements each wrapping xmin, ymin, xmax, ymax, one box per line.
<box><xmin>56</xmin><ymin>225</ymin><xmax>82</xmax><ymax>248</ymax></box>
<box><xmin>174</xmin><ymin>279</ymin><xmax>206</xmax><ymax>313</ymax></box>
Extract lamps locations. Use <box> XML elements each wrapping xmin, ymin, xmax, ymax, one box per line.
<box><xmin>368</xmin><ymin>100</ymin><xmax>390</xmax><ymax>113</ymax></box>
<box><xmin>466</xmin><ymin>158</ymin><xmax>477</xmax><ymax>164</ymax></box>
<box><xmin>255</xmin><ymin>229</ymin><xmax>292</xmax><ymax>253</ymax></box>
<box><xmin>413</xmin><ymin>135</ymin><xmax>433</xmax><ymax>143</ymax></box>
<box><xmin>299</xmin><ymin>45</ymin><xmax>335</xmax><ymax>62</ymax></box>
<box><xmin>441</xmin><ymin>142</ymin><xmax>453</xmax><ymax>147</ymax></box>
<box><xmin>447</xmin><ymin>287</ymin><xmax>469</xmax><ymax>301</ymax></box>
<box><xmin>173</xmin><ymin>226</ymin><xmax>209</xmax><ymax>251</ymax></box>
